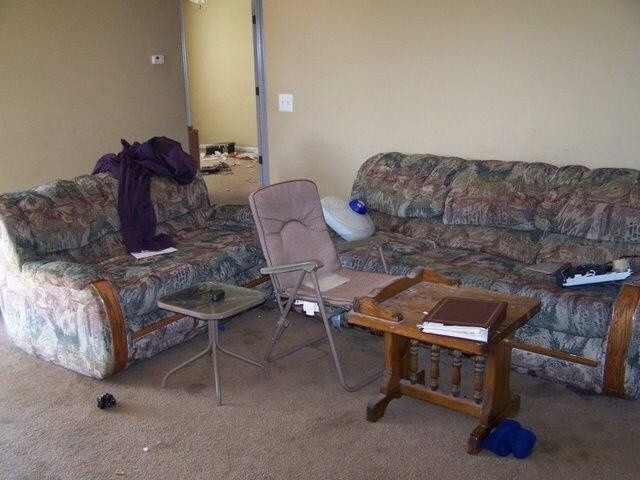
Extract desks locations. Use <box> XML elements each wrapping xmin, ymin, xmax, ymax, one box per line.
<box><xmin>157</xmin><ymin>281</ymin><xmax>266</xmax><ymax>406</ymax></box>
<box><xmin>346</xmin><ymin>265</ymin><xmax>541</xmax><ymax>455</ymax></box>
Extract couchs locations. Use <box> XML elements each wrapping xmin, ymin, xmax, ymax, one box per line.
<box><xmin>296</xmin><ymin>151</ymin><xmax>640</xmax><ymax>400</ymax></box>
<box><xmin>0</xmin><ymin>164</ymin><xmax>280</xmax><ymax>378</ymax></box>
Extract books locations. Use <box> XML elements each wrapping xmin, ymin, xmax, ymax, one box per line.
<box><xmin>417</xmin><ymin>296</ymin><xmax>508</xmax><ymax>343</ymax></box>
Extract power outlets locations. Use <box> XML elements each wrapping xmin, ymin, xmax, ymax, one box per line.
<box><xmin>278</xmin><ymin>94</ymin><xmax>293</xmax><ymax>112</ymax></box>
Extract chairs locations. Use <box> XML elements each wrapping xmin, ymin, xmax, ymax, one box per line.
<box><xmin>248</xmin><ymin>179</ymin><xmax>401</xmax><ymax>393</ymax></box>
